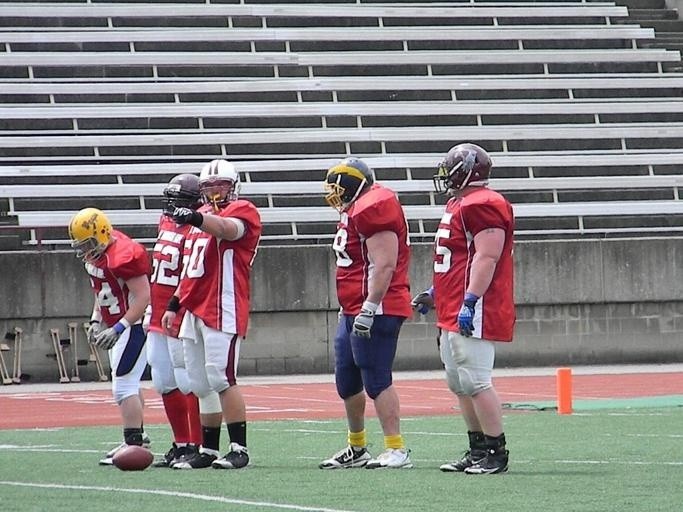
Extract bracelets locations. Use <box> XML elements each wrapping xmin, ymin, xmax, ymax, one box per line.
<box><xmin>166</xmin><ymin>296</ymin><xmax>179</xmax><ymax>313</ymax></box>
<box><xmin>90</xmin><ymin>311</ymin><xmax>103</xmax><ymax>323</ymax></box>
<box><xmin>190</xmin><ymin>211</ymin><xmax>203</xmax><ymax>227</ymax></box>
<box><xmin>362</xmin><ymin>302</ymin><xmax>378</xmax><ymax>313</ymax></box>
<box><xmin>113</xmin><ymin>322</ymin><xmax>126</xmax><ymax>334</ymax></box>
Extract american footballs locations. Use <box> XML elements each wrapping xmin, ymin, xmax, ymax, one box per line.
<box><xmin>112</xmin><ymin>445</ymin><xmax>154</xmax><ymax>471</ymax></box>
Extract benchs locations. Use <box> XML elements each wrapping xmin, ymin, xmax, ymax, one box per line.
<box><xmin>0</xmin><ymin>0</ymin><xmax>683</xmax><ymax>249</ymax></box>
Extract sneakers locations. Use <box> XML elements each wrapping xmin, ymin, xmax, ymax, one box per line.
<box><xmin>318</xmin><ymin>445</ymin><xmax>372</xmax><ymax>469</ymax></box>
<box><xmin>366</xmin><ymin>448</ymin><xmax>413</xmax><ymax>469</ymax></box>
<box><xmin>153</xmin><ymin>442</ymin><xmax>248</xmax><ymax>469</ymax></box>
<box><xmin>440</xmin><ymin>450</ymin><xmax>509</xmax><ymax>473</ymax></box>
<box><xmin>99</xmin><ymin>430</ymin><xmax>152</xmax><ymax>465</ymax></box>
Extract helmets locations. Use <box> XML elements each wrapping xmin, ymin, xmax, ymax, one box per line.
<box><xmin>198</xmin><ymin>160</ymin><xmax>241</xmax><ymax>212</ymax></box>
<box><xmin>325</xmin><ymin>157</ymin><xmax>373</xmax><ymax>214</ymax></box>
<box><xmin>433</xmin><ymin>143</ymin><xmax>492</xmax><ymax>197</ymax></box>
<box><xmin>160</xmin><ymin>174</ymin><xmax>204</xmax><ymax>223</ymax></box>
<box><xmin>68</xmin><ymin>208</ymin><xmax>114</xmax><ymax>264</ymax></box>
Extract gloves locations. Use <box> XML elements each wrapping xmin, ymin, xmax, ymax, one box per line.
<box><xmin>352</xmin><ymin>301</ymin><xmax>377</xmax><ymax>339</ymax></box>
<box><xmin>410</xmin><ymin>288</ymin><xmax>435</xmax><ymax>314</ymax></box>
<box><xmin>164</xmin><ymin>204</ymin><xmax>203</xmax><ymax>227</ymax></box>
<box><xmin>458</xmin><ymin>293</ymin><xmax>478</xmax><ymax>338</ymax></box>
<box><xmin>87</xmin><ymin>322</ymin><xmax>120</xmax><ymax>350</ymax></box>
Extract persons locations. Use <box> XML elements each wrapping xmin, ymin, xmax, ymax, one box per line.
<box><xmin>162</xmin><ymin>159</ymin><xmax>262</xmax><ymax>470</ymax></box>
<box><xmin>68</xmin><ymin>207</ymin><xmax>151</xmax><ymax>465</ymax></box>
<box><xmin>409</xmin><ymin>142</ymin><xmax>516</xmax><ymax>473</ymax></box>
<box><xmin>143</xmin><ymin>173</ymin><xmax>203</xmax><ymax>468</ymax></box>
<box><xmin>319</xmin><ymin>157</ymin><xmax>414</xmax><ymax>470</ymax></box>
